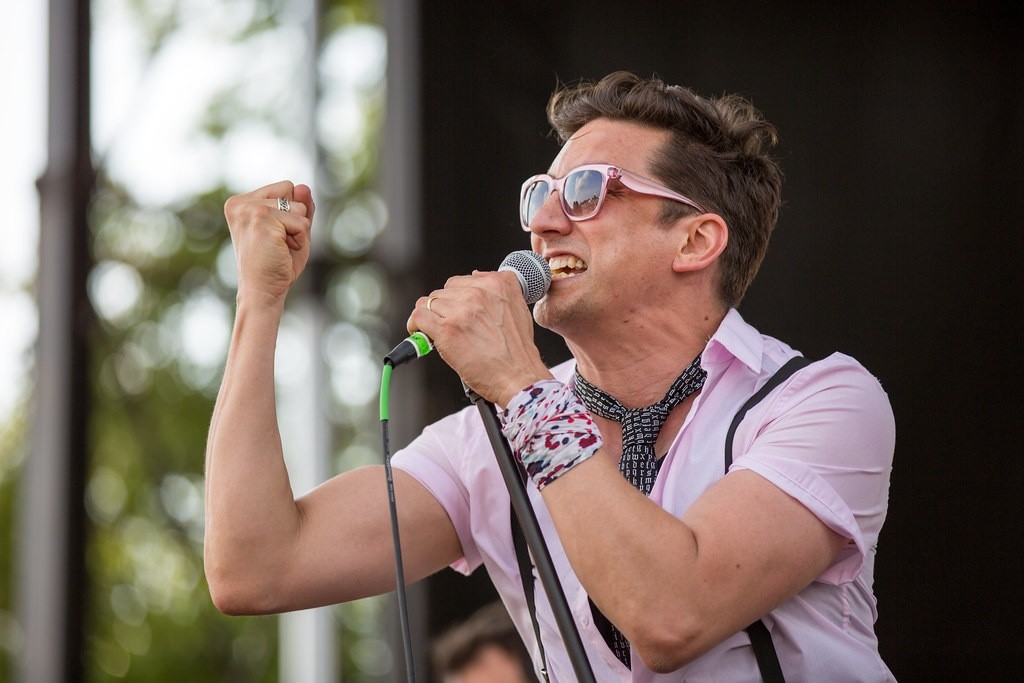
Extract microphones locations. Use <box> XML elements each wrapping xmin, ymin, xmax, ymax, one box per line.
<box><xmin>383</xmin><ymin>250</ymin><xmax>552</xmax><ymax>369</ymax></box>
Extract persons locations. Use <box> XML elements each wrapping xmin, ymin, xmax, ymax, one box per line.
<box><xmin>427</xmin><ymin>599</ymin><xmax>540</xmax><ymax>683</ymax></box>
<box><xmin>201</xmin><ymin>72</ymin><xmax>900</xmax><ymax>683</ymax></box>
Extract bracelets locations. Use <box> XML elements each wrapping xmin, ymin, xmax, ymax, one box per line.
<box><xmin>496</xmin><ymin>381</ymin><xmax>605</xmax><ymax>491</ymax></box>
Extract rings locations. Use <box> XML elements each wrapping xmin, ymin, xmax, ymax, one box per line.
<box><xmin>427</xmin><ymin>296</ymin><xmax>439</xmax><ymax>310</ymax></box>
<box><xmin>277</xmin><ymin>197</ymin><xmax>290</xmax><ymax>211</ymax></box>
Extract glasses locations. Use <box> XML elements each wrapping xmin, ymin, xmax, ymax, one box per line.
<box><xmin>519</xmin><ymin>162</ymin><xmax>709</xmax><ymax>233</ymax></box>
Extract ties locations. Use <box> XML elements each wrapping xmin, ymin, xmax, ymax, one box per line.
<box><xmin>572</xmin><ymin>347</ymin><xmax>708</xmax><ymax>673</ymax></box>
